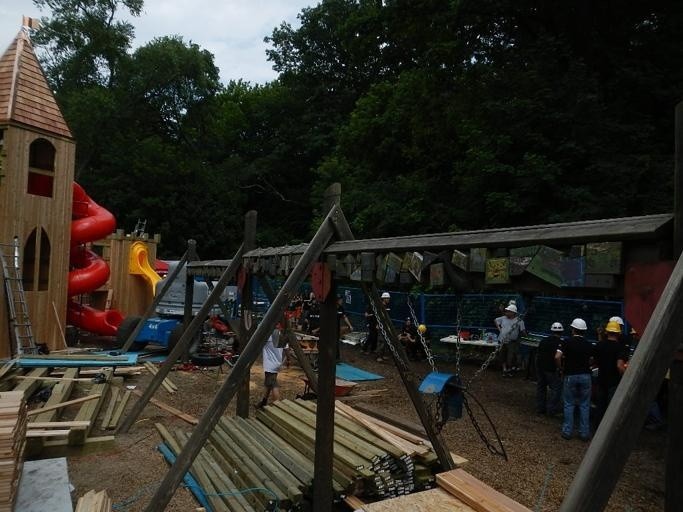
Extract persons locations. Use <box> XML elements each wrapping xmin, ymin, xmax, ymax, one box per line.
<box><xmin>334</xmin><ymin>294</ymin><xmax>353</xmax><ymax>364</ymax></box>
<box><xmin>300</xmin><ymin>297</ymin><xmax>321</xmax><ymax>372</ymax></box>
<box><xmin>252</xmin><ymin>323</ymin><xmax>290</xmax><ymax>409</ymax></box>
<box><xmin>300</xmin><ymin>292</ymin><xmax>316</xmax><ymax>333</ymax></box>
<box><xmin>373</xmin><ymin>290</ymin><xmax>394</xmax><ymax>363</ymax></box>
<box><xmin>406</xmin><ymin>324</ymin><xmax>432</xmax><ymax>360</ymax></box>
<box><xmin>396</xmin><ymin>316</ymin><xmax>419</xmax><ymax>357</ymax></box>
<box><xmin>628</xmin><ymin>328</ymin><xmax>641</xmax><ymax>345</ymax></box>
<box><xmin>553</xmin><ymin>318</ymin><xmax>598</xmax><ymax>441</ymax></box>
<box><xmin>592</xmin><ymin>322</ymin><xmax>628</xmax><ymax>412</ymax></box>
<box><xmin>504</xmin><ymin>282</ymin><xmax>527</xmax><ymax>372</ymax></box>
<box><xmin>358</xmin><ymin>304</ymin><xmax>378</xmax><ymax>355</ymax></box>
<box><xmin>607</xmin><ymin>315</ymin><xmax>630</xmax><ymax>365</ymax></box>
<box><xmin>495</xmin><ymin>299</ymin><xmax>533</xmax><ymax>378</ymax></box>
<box><xmin>532</xmin><ymin>321</ymin><xmax>565</xmax><ymax>418</ymax></box>
<box><xmin>645</xmin><ymin>401</ymin><xmax>663</xmax><ymax>430</ymax></box>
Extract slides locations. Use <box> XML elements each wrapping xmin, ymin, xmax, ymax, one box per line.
<box><xmin>69</xmin><ymin>181</ymin><xmax>126</xmax><ymax>337</ymax></box>
<box><xmin>128</xmin><ymin>241</ymin><xmax>163</xmax><ymax>298</ymax></box>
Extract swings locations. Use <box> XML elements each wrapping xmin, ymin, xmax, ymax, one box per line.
<box><xmin>191</xmin><ymin>288</ymin><xmax>225</xmax><ymax>367</ymax></box>
<box><xmin>366</xmin><ymin>282</ymin><xmax>529</xmax><ymax>460</ymax></box>
<box><xmin>273</xmin><ymin>269</ymin><xmax>302</xmax><ymax>349</ymax></box>
<box><xmin>241</xmin><ymin>264</ymin><xmax>259</xmax><ymax>333</ymax></box>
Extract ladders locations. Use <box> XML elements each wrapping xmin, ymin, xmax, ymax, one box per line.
<box><xmin>0</xmin><ymin>235</ymin><xmax>39</xmax><ymax>356</ymax></box>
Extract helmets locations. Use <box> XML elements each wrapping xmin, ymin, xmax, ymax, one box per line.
<box><xmin>336</xmin><ymin>294</ymin><xmax>342</xmax><ymax>301</ymax></box>
<box><xmin>505</xmin><ymin>304</ymin><xmax>516</xmax><ymax>312</ymax></box>
<box><xmin>605</xmin><ymin>317</ymin><xmax>624</xmax><ymax>333</ymax></box>
<box><xmin>380</xmin><ymin>293</ymin><xmax>391</xmax><ymax>299</ymax></box>
<box><xmin>405</xmin><ymin>316</ymin><xmax>410</xmax><ymax>321</ymax></box>
<box><xmin>570</xmin><ymin>318</ymin><xmax>587</xmax><ymax>330</ymax></box>
<box><xmin>550</xmin><ymin>323</ymin><xmax>563</xmax><ymax>331</ymax></box>
<box><xmin>418</xmin><ymin>325</ymin><xmax>425</xmax><ymax>333</ymax></box>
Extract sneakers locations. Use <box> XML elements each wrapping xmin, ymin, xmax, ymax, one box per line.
<box><xmin>367</xmin><ymin>354</ymin><xmax>388</xmax><ymax>362</ymax></box>
<box><xmin>562</xmin><ymin>433</ymin><xmax>589</xmax><ymax>440</ymax></box>
<box><xmin>538</xmin><ymin>410</ymin><xmax>561</xmax><ymax>417</ymax></box>
<box><xmin>254</xmin><ymin>401</ymin><xmax>263</xmax><ymax>407</ymax></box>
<box><xmin>502</xmin><ymin>366</ymin><xmax>520</xmax><ymax>376</ymax></box>
<box><xmin>645</xmin><ymin>417</ymin><xmax>661</xmax><ymax>431</ymax></box>
